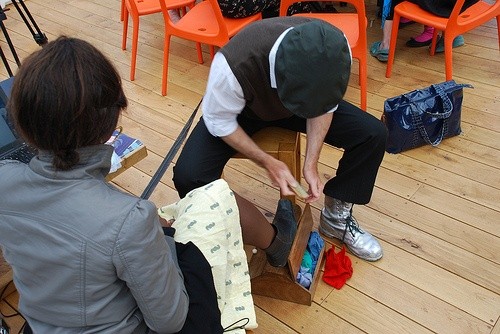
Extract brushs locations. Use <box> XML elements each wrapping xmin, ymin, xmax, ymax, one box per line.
<box><xmin>289</xmin><ymin>183</ymin><xmax>310</xmax><ymax>199</ymax></box>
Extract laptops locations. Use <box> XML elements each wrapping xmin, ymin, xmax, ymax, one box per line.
<box><xmin>0</xmin><ymin>86</ymin><xmax>39</xmax><ymax>164</ymax></box>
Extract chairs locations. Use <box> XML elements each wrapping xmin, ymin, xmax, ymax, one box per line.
<box><xmin>386</xmin><ymin>0</ymin><xmax>500</xmax><ymax>81</ymax></box>
<box><xmin>280</xmin><ymin>0</ymin><xmax>367</xmax><ymax>112</ymax></box>
<box><xmin>121</xmin><ymin>0</ymin><xmax>262</xmax><ymax>96</ymax></box>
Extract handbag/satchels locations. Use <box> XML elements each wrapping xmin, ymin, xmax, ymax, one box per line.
<box><xmin>379</xmin><ymin>79</ymin><xmax>474</xmax><ymax>154</ymax></box>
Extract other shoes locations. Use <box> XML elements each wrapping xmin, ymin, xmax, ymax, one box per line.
<box><xmin>405</xmin><ymin>35</ymin><xmax>439</xmax><ymax>48</ymax></box>
<box><xmin>435</xmin><ymin>34</ymin><xmax>465</xmax><ymax>53</ymax></box>
<box><xmin>370</xmin><ymin>41</ymin><xmax>389</xmax><ymax>62</ymax></box>
<box><xmin>398</xmin><ymin>20</ymin><xmax>415</xmax><ymax>29</ymax></box>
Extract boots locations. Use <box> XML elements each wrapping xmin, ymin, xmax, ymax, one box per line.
<box><xmin>317</xmin><ymin>195</ymin><xmax>384</xmax><ymax>263</ymax></box>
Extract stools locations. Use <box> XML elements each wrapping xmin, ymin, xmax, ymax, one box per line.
<box><xmin>220</xmin><ymin>126</ymin><xmax>300</xmax><ymax>203</ymax></box>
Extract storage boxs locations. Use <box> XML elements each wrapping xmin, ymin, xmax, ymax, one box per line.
<box><xmin>248</xmin><ymin>203</ymin><xmax>327</xmax><ymax>306</ymax></box>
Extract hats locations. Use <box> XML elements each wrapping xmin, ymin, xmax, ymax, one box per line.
<box><xmin>275</xmin><ymin>21</ymin><xmax>352</xmax><ymax>119</ymax></box>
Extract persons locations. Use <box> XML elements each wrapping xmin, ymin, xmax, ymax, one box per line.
<box><xmin>171</xmin><ymin>17</ymin><xmax>390</xmax><ymax>268</ymax></box>
<box><xmin>0</xmin><ymin>34</ymin><xmax>225</xmax><ymax>334</ymax></box>
<box><xmin>370</xmin><ymin>0</ymin><xmax>480</xmax><ymax>63</ymax></box>
<box><xmin>216</xmin><ymin>0</ymin><xmax>338</xmax><ymax>20</ymax></box>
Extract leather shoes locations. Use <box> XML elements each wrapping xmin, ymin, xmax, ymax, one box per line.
<box><xmin>263</xmin><ymin>199</ymin><xmax>297</xmax><ymax>268</ymax></box>
